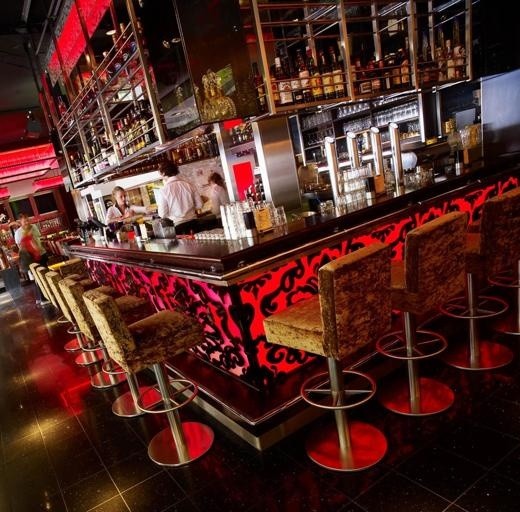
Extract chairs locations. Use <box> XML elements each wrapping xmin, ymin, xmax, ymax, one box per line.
<box><xmin>450</xmin><ymin>188</ymin><xmax>520</xmax><ymax>337</ymax></box>
<box><xmin>45</xmin><ymin>272</ymin><xmax>99</xmax><ymax>364</ymax></box>
<box><xmin>59</xmin><ymin>278</ymin><xmax>148</xmax><ymax>420</ymax></box>
<box><xmin>262</xmin><ymin>242</ymin><xmax>392</xmax><ymax>471</ymax></box>
<box><xmin>82</xmin><ymin>288</ymin><xmax>214</xmax><ymax>469</ymax></box>
<box><xmin>28</xmin><ymin>257</ymin><xmax>79</xmax><ymax>324</ymax></box>
<box><xmin>378</xmin><ymin>210</ymin><xmax>471</xmax><ymax>417</ymax></box>
<box><xmin>37</xmin><ymin>266</ymin><xmax>87</xmax><ymax>350</ymax></box>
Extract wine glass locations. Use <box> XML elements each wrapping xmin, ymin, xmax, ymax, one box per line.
<box><xmin>306</xmin><ymin>127</ymin><xmax>334</xmax><ymax>145</ymax></box>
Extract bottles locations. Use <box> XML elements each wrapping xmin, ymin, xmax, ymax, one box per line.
<box><xmin>167</xmin><ymin>136</ymin><xmax>216</xmax><ymax>163</ymax></box>
<box><xmin>101</xmin><ymin>19</ymin><xmax>138</xmax><ymax>84</ymax></box>
<box><xmin>69</xmin><ymin>151</ymin><xmax>91</xmax><ymax>184</ymax></box>
<box><xmin>87</xmin><ymin>136</ymin><xmax>119</xmax><ymax>175</ymax></box>
<box><xmin>71</xmin><ymin>71</ymin><xmax>98</xmax><ymax>115</ymax></box>
<box><xmin>269</xmin><ymin>51</ymin><xmax>345</xmax><ymax>108</ymax></box>
<box><xmin>351</xmin><ymin>50</ymin><xmax>411</xmax><ymax>93</ymax></box>
<box><xmin>114</xmin><ymin>100</ymin><xmax>158</xmax><ymax>158</ymax></box>
<box><xmin>253</xmin><ymin>59</ymin><xmax>268</xmax><ymax>110</ymax></box>
<box><xmin>454</xmin><ymin>145</ymin><xmax>465</xmax><ymax>178</ymax></box>
<box><xmin>136</xmin><ymin>20</ymin><xmax>148</xmax><ymax>50</ymax></box>
<box><xmin>57</xmin><ymin>96</ymin><xmax>71</xmax><ymax>126</ymax></box>
<box><xmin>414</xmin><ymin>39</ymin><xmax>468</xmax><ymax>86</ymax></box>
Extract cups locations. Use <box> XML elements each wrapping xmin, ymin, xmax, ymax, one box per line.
<box><xmin>336</xmin><ymin>103</ymin><xmax>370</xmax><ymax>119</ymax></box>
<box><xmin>301</xmin><ymin>112</ymin><xmax>334</xmax><ymax>127</ymax></box>
<box><xmin>254</xmin><ymin>198</ymin><xmax>287</xmax><ymax>232</ymax></box>
<box><xmin>371</xmin><ymin>102</ymin><xmax>418</xmax><ymax>126</ymax></box>
<box><xmin>340</xmin><ymin>116</ymin><xmax>372</xmax><ymax>134</ymax></box>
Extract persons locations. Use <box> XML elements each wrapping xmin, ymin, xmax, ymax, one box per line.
<box><xmin>298</xmin><ymin>156</ymin><xmax>316</xmax><ymax>190</ymax></box>
<box><xmin>14</xmin><ymin>211</ymin><xmax>48</xmax><ymax>271</ymax></box>
<box><xmin>208</xmin><ymin>172</ymin><xmax>228</xmax><ymax>219</ymax></box>
<box><xmin>20</xmin><ymin>224</ymin><xmax>51</xmax><ymax>306</ymax></box>
<box><xmin>106</xmin><ymin>186</ymin><xmax>146</xmax><ymax>230</ymax></box>
<box><xmin>155</xmin><ymin>161</ymin><xmax>204</xmax><ymax>237</ymax></box>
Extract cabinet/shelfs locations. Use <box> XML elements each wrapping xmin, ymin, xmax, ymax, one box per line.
<box><xmin>370</xmin><ymin>89</ymin><xmax>425</xmax><ymax>143</ymax></box>
<box><xmin>299</xmin><ymin>111</ymin><xmax>340</xmax><ymax>158</ymax></box>
<box><xmin>242</xmin><ymin>0</ymin><xmax>474</xmax><ymax>116</ymax></box>
<box><xmin>86</xmin><ymin>112</ymin><xmax>300</xmax><ymax>222</ymax></box>
<box><xmin>35</xmin><ymin>1</ymin><xmax>203</xmax><ymax>188</ymax></box>
<box><xmin>1</xmin><ymin>209</ymin><xmax>61</xmax><ymax>244</ymax></box>
<box><xmin>330</xmin><ymin>100</ymin><xmax>371</xmax><ymax>153</ymax></box>
